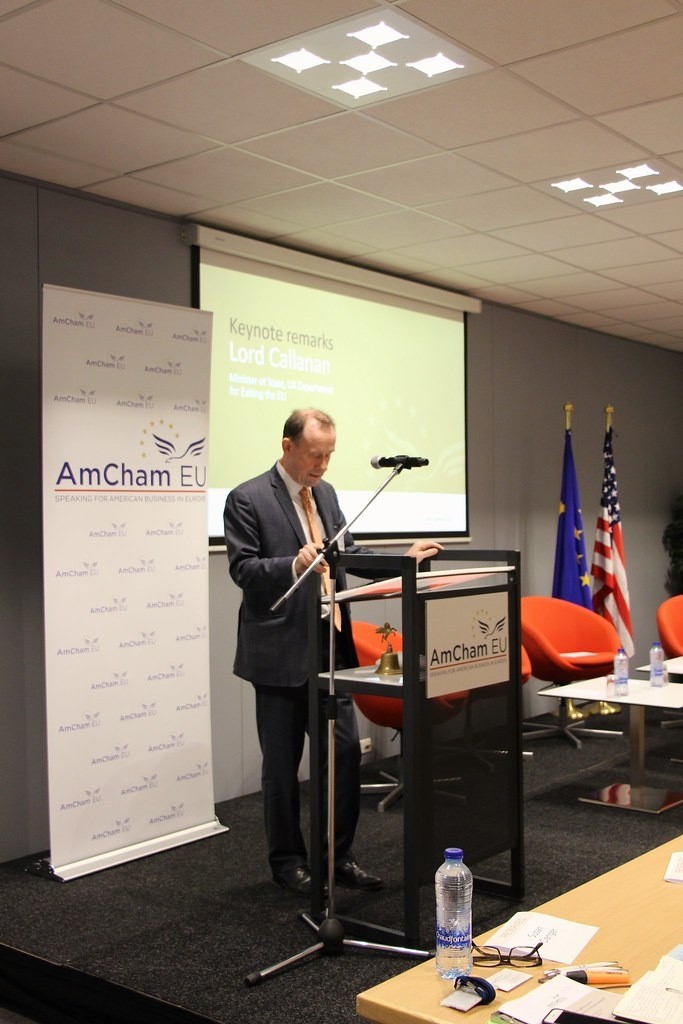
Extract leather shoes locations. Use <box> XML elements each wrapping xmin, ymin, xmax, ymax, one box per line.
<box><xmin>273</xmin><ymin>866</ymin><xmax>328</xmax><ymax>897</ymax></box>
<box><xmin>333</xmin><ymin>861</ymin><xmax>384</xmax><ymax>889</ymax></box>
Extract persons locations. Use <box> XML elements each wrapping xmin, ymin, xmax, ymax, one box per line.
<box><xmin>223</xmin><ymin>408</ymin><xmax>444</xmax><ymax>891</ymax></box>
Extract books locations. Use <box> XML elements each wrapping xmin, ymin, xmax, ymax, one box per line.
<box><xmin>489</xmin><ymin>942</ymin><xmax>683</xmax><ymax>1024</ymax></box>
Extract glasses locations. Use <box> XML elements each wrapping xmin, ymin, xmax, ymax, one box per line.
<box><xmin>470</xmin><ymin>940</ymin><xmax>544</xmax><ymax>967</ymax></box>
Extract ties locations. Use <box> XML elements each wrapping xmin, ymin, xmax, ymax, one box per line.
<box><xmin>299</xmin><ymin>487</ymin><xmax>342</xmax><ymax>633</ymax></box>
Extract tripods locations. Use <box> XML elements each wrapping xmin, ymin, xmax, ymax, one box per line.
<box><xmin>245</xmin><ymin>467</ymin><xmax>440</xmax><ymax>984</ymax></box>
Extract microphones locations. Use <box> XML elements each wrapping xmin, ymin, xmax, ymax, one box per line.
<box><xmin>370</xmin><ymin>455</ymin><xmax>429</xmax><ymax>469</ymax></box>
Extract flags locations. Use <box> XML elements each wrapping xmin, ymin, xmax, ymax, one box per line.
<box><xmin>590</xmin><ymin>428</ymin><xmax>636</xmax><ymax>659</ymax></box>
<box><xmin>552</xmin><ymin>430</ymin><xmax>593</xmax><ymax>612</ymax></box>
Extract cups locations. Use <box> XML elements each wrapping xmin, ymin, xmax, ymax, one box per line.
<box><xmin>663</xmin><ymin>664</ymin><xmax>668</xmax><ymax>685</ymax></box>
<box><xmin>607</xmin><ymin>674</ymin><xmax>620</xmax><ymax>698</ymax></box>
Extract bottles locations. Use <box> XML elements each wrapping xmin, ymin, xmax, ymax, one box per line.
<box><xmin>649</xmin><ymin>642</ymin><xmax>664</xmax><ymax>687</ymax></box>
<box><xmin>435</xmin><ymin>848</ymin><xmax>473</xmax><ymax>980</ymax></box>
<box><xmin>613</xmin><ymin>648</ymin><xmax>628</xmax><ymax>696</ymax></box>
